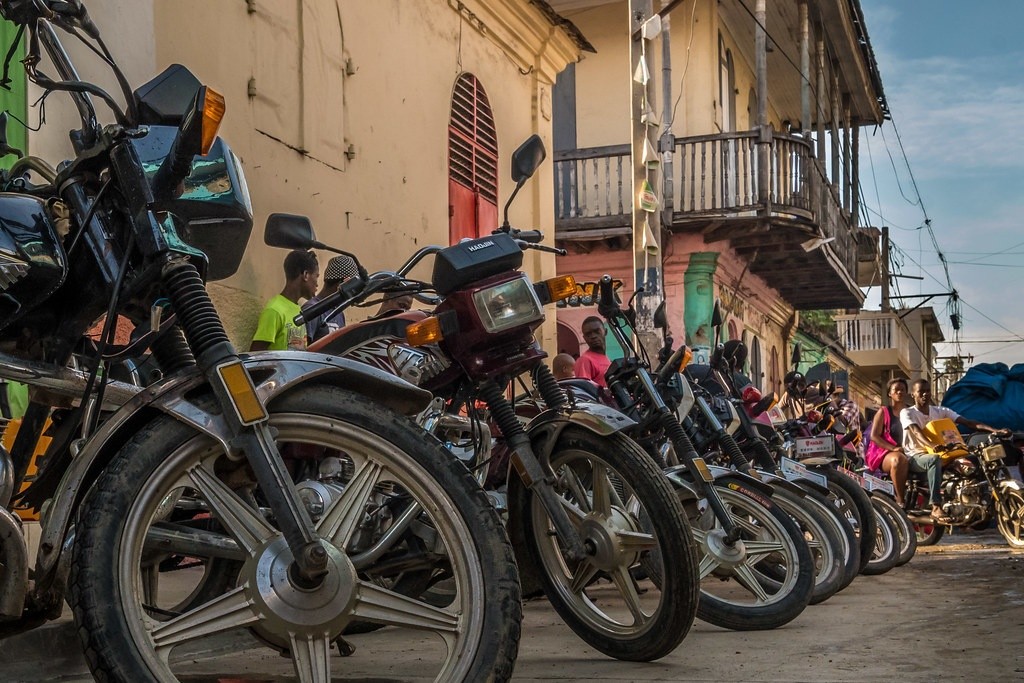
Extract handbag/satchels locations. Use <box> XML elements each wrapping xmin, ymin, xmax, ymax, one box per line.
<box><xmin>865</xmin><ymin>406</ymin><xmax>897</xmax><ymax>472</ymax></box>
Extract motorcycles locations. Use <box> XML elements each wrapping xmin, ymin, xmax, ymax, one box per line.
<box><xmin>229</xmin><ymin>272</ymin><xmax>1024</xmax><ymax>683</ymax></box>
<box><xmin>147</xmin><ymin>132</ymin><xmax>703</xmax><ymax>664</ymax></box>
<box><xmin>0</xmin><ymin>1</ymin><xmax>525</xmax><ymax>683</ymax></box>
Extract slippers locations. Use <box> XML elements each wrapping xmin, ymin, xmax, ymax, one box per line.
<box><xmin>929</xmin><ymin>512</ymin><xmax>956</xmax><ymax>522</ymax></box>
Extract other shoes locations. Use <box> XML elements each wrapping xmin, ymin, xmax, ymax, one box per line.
<box><xmin>895</xmin><ymin>499</ymin><xmax>906</xmax><ymax>509</ymax></box>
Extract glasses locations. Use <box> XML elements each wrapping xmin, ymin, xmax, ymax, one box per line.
<box><xmin>302</xmin><ymin>251</ymin><xmax>317</xmax><ymax>274</ymax></box>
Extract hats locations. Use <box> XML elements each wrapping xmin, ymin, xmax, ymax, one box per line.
<box><xmin>811</xmin><ymin>380</ymin><xmax>843</xmax><ymax>394</ymax></box>
<box><xmin>324</xmin><ymin>255</ymin><xmax>361</xmax><ymax>279</ymax></box>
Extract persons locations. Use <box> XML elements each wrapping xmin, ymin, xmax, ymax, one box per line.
<box><xmin>784</xmin><ymin>371</ymin><xmax>870</xmax><ymax>449</ymax></box>
<box><xmin>870</xmin><ymin>378</ymin><xmax>910</xmax><ymax>509</ymax></box>
<box><xmin>554</xmin><ymin>354</ymin><xmax>575</xmax><ymax>378</ymax></box>
<box><xmin>375</xmin><ymin>289</ymin><xmax>412</xmax><ymax>313</ymax></box>
<box><xmin>250</xmin><ymin>255</ymin><xmax>320</xmax><ymax>485</ymax></box>
<box><xmin>301</xmin><ymin>256</ymin><xmax>358</xmax><ymax>343</ymax></box>
<box><xmin>577</xmin><ymin>316</ymin><xmax>612</xmax><ymax>389</ymax></box>
<box><xmin>722</xmin><ymin>340</ymin><xmax>752</xmax><ymax>390</ymax></box>
<box><xmin>900</xmin><ymin>379</ymin><xmax>1010</xmax><ymax>523</ymax></box>
